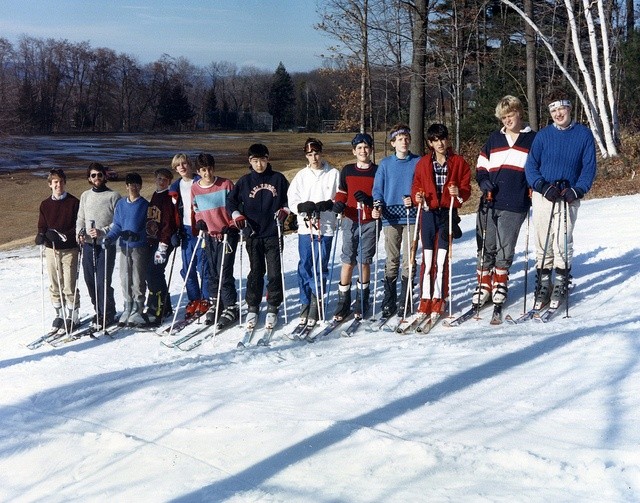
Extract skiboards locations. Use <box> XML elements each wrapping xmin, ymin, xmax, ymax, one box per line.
<box><xmin>51</xmin><ymin>312</ymin><xmax>124</xmax><ymax>347</ymax></box>
<box><xmin>162</xmin><ymin>314</ymin><xmax>199</xmax><ymax>335</ymax></box>
<box><xmin>397</xmin><ymin>301</ymin><xmax>449</xmax><ymax>334</ymax></box>
<box><xmin>160</xmin><ymin>299</ymin><xmax>248</xmax><ymax>351</ymax></box>
<box><xmin>306</xmin><ymin>296</ymin><xmax>376</xmax><ymax>343</ymax></box>
<box><xmin>505</xmin><ymin>284</ymin><xmax>576</xmax><ymax>325</ymax></box>
<box><xmin>442</xmin><ymin>304</ymin><xmax>504</xmax><ymax>327</ymax></box>
<box><xmin>90</xmin><ymin>326</ymin><xmax>124</xmax><ymax>340</ymax></box>
<box><xmin>283</xmin><ymin>302</ymin><xmax>328</xmax><ymax>341</ymax></box>
<box><xmin>24</xmin><ymin>314</ymin><xmax>96</xmax><ymax>349</ymax></box>
<box><xmin>237</xmin><ymin>305</ymin><xmax>272</xmax><ymax>349</ymax></box>
<box><xmin>365</xmin><ymin>292</ymin><xmax>419</xmax><ymax>332</ymax></box>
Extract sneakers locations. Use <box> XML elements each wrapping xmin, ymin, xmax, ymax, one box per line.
<box><xmin>246</xmin><ymin>306</ymin><xmax>259</xmax><ymax>328</ymax></box>
<box><xmin>417</xmin><ymin>298</ymin><xmax>431</xmax><ymax>315</ymax></box>
<box><xmin>265</xmin><ymin>304</ymin><xmax>278</xmax><ymax>329</ymax></box>
<box><xmin>89</xmin><ymin>313</ymin><xmax>117</xmax><ymax>330</ymax></box>
<box><xmin>431</xmin><ymin>298</ymin><xmax>446</xmax><ymax>315</ymax></box>
<box><xmin>195</xmin><ymin>299</ymin><xmax>212</xmax><ymax>315</ymax></box>
<box><xmin>218</xmin><ymin>304</ymin><xmax>238</xmax><ymax>326</ymax></box>
<box><xmin>185</xmin><ymin>299</ymin><xmax>201</xmax><ymax>316</ymax></box>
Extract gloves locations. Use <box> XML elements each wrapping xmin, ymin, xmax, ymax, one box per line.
<box><xmin>46</xmin><ymin>230</ymin><xmax>59</xmax><ymax>241</ymax></box>
<box><xmin>315</xmin><ymin>199</ymin><xmax>334</xmax><ymax>213</ymax></box>
<box><xmin>560</xmin><ymin>187</ymin><xmax>581</xmax><ymax>204</ymax></box>
<box><xmin>480</xmin><ymin>178</ymin><xmax>495</xmax><ymax>194</ymax></box>
<box><xmin>535</xmin><ymin>178</ymin><xmax>560</xmax><ymax>201</ymax></box>
<box><xmin>274</xmin><ymin>207</ymin><xmax>290</xmax><ymax>223</ymax></box>
<box><xmin>298</xmin><ymin>201</ymin><xmax>315</xmax><ymax>214</ymax></box>
<box><xmin>121</xmin><ymin>230</ymin><xmax>138</xmax><ymax>242</ymax></box>
<box><xmin>171</xmin><ymin>233</ymin><xmax>180</xmax><ymax>247</ymax></box>
<box><xmin>154</xmin><ymin>241</ymin><xmax>168</xmax><ymax>265</ymax></box>
<box><xmin>196</xmin><ymin>219</ymin><xmax>207</xmax><ymax>232</ymax></box>
<box><xmin>440</xmin><ymin>215</ymin><xmax>449</xmax><ymax>242</ymax></box>
<box><xmin>452</xmin><ymin>216</ymin><xmax>462</xmax><ymax>238</ymax></box>
<box><xmin>354</xmin><ymin>191</ymin><xmax>373</xmax><ymax>208</ymax></box>
<box><xmin>102</xmin><ymin>236</ymin><xmax>111</xmax><ymax>249</ymax></box>
<box><xmin>222</xmin><ymin>226</ymin><xmax>237</xmax><ymax>235</ymax></box>
<box><xmin>231</xmin><ymin>211</ymin><xmax>247</xmax><ymax>229</ymax></box>
<box><xmin>35</xmin><ymin>233</ymin><xmax>44</xmax><ymax>244</ymax></box>
<box><xmin>332</xmin><ymin>201</ymin><xmax>346</xmax><ymax>213</ymax></box>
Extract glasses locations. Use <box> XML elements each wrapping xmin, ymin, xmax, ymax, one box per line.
<box><xmin>353</xmin><ymin>133</ymin><xmax>373</xmax><ymax>147</ymax></box>
<box><xmin>305</xmin><ymin>141</ymin><xmax>321</xmax><ymax>153</ymax></box>
<box><xmin>91</xmin><ymin>173</ymin><xmax>102</xmax><ymax>178</ymax></box>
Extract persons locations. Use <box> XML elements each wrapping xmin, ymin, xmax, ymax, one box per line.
<box><xmin>34</xmin><ymin>165</ymin><xmax>81</xmax><ymax>336</ymax></box>
<box><xmin>524</xmin><ymin>90</ymin><xmax>597</xmax><ymax>303</ymax></box>
<box><xmin>75</xmin><ymin>163</ymin><xmax>121</xmax><ymax>331</ymax></box>
<box><xmin>170</xmin><ymin>151</ymin><xmax>210</xmax><ymax>319</ymax></box>
<box><xmin>471</xmin><ymin>94</ymin><xmax>538</xmax><ymax>306</ymax></box>
<box><xmin>286</xmin><ymin>137</ymin><xmax>340</xmax><ymax>325</ymax></box>
<box><xmin>143</xmin><ymin>168</ymin><xmax>174</xmax><ymax>317</ymax></box>
<box><xmin>331</xmin><ymin>133</ymin><xmax>382</xmax><ymax>318</ymax></box>
<box><xmin>107</xmin><ymin>173</ymin><xmax>148</xmax><ymax>326</ymax></box>
<box><xmin>191</xmin><ymin>152</ymin><xmax>244</xmax><ymax>322</ymax></box>
<box><xmin>226</xmin><ymin>143</ymin><xmax>290</xmax><ymax>330</ymax></box>
<box><xmin>411</xmin><ymin>122</ymin><xmax>471</xmax><ymax>315</ymax></box>
<box><xmin>372</xmin><ymin>122</ymin><xmax>422</xmax><ymax>319</ymax></box>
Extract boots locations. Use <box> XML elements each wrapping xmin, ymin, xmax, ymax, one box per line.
<box><xmin>118</xmin><ymin>300</ymin><xmax>132</xmax><ymax>325</ymax></box>
<box><xmin>307</xmin><ymin>293</ymin><xmax>322</xmax><ymax>327</ymax></box>
<box><xmin>332</xmin><ymin>281</ymin><xmax>351</xmax><ymax>323</ymax></box>
<box><xmin>142</xmin><ymin>288</ymin><xmax>173</xmax><ymax>324</ymax></box>
<box><xmin>48</xmin><ymin>303</ymin><xmax>66</xmax><ymax>332</ymax></box>
<box><xmin>471</xmin><ymin>268</ymin><xmax>492</xmax><ymax>305</ymax></box>
<box><xmin>397</xmin><ymin>275</ymin><xmax>415</xmax><ymax>317</ymax></box>
<box><xmin>299</xmin><ymin>303</ymin><xmax>308</xmax><ymax>325</ymax></box>
<box><xmin>550</xmin><ymin>267</ymin><xmax>572</xmax><ymax>304</ymax></box>
<box><xmin>127</xmin><ymin>302</ymin><xmax>143</xmax><ymax>326</ymax></box>
<box><xmin>535</xmin><ymin>267</ymin><xmax>553</xmax><ymax>305</ymax></box>
<box><xmin>57</xmin><ymin>302</ymin><xmax>80</xmax><ymax>335</ymax></box>
<box><xmin>206</xmin><ymin>297</ymin><xmax>222</xmax><ymax>321</ymax></box>
<box><xmin>491</xmin><ymin>266</ymin><xmax>509</xmax><ymax>304</ymax></box>
<box><xmin>354</xmin><ymin>279</ymin><xmax>370</xmax><ymax>319</ymax></box>
<box><xmin>382</xmin><ymin>276</ymin><xmax>398</xmax><ymax>316</ymax></box>
